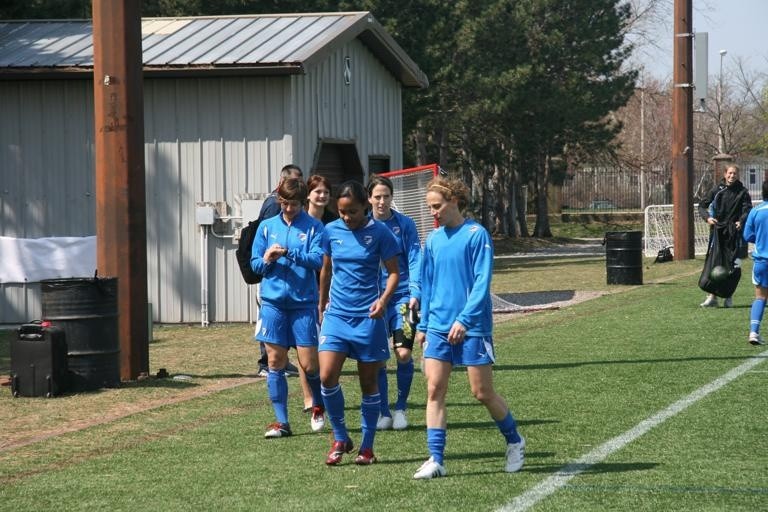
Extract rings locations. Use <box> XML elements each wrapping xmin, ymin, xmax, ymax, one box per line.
<box><xmin>458</xmin><ymin>333</ymin><xmax>462</xmax><ymax>337</ymax></box>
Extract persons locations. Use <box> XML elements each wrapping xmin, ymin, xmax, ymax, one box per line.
<box><xmin>303</xmin><ymin>173</ymin><xmax>333</xmax><ymax>224</ymax></box>
<box><xmin>743</xmin><ymin>180</ymin><xmax>768</xmax><ymax>346</ymax></box>
<box><xmin>256</xmin><ymin>163</ymin><xmax>303</xmax><ymax>378</ymax></box>
<box><xmin>697</xmin><ymin>162</ymin><xmax>752</xmax><ymax>308</ymax></box>
<box><xmin>249</xmin><ymin>177</ymin><xmax>323</xmax><ymax>438</ymax></box>
<box><xmin>315</xmin><ymin>180</ymin><xmax>401</xmax><ymax>465</ymax></box>
<box><xmin>362</xmin><ymin>176</ymin><xmax>423</xmax><ymax>431</ymax></box>
<box><xmin>406</xmin><ymin>171</ymin><xmax>527</xmax><ymax>482</ymax></box>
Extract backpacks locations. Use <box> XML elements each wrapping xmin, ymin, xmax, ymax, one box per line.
<box><xmin>233</xmin><ymin>202</ymin><xmax>275</xmax><ymax>286</ymax></box>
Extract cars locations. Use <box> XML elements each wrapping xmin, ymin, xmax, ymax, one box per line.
<box><xmin>588</xmin><ymin>200</ymin><xmax>617</xmax><ymax>209</ymax></box>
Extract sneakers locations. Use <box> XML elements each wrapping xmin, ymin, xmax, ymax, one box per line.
<box><xmin>502</xmin><ymin>433</ymin><xmax>527</xmax><ymax>474</ymax></box>
<box><xmin>723</xmin><ymin>297</ymin><xmax>734</xmax><ymax>308</ymax></box>
<box><xmin>412</xmin><ymin>456</ymin><xmax>447</xmax><ymax>480</ymax></box>
<box><xmin>324</xmin><ymin>437</ymin><xmax>356</xmax><ymax>466</ymax></box>
<box><xmin>310</xmin><ymin>405</ymin><xmax>327</xmax><ymax>432</ymax></box>
<box><xmin>263</xmin><ymin>422</ymin><xmax>293</xmax><ymax>439</ymax></box>
<box><xmin>374</xmin><ymin>410</ymin><xmax>409</xmax><ymax>431</ymax></box>
<box><xmin>354</xmin><ymin>449</ymin><xmax>378</xmax><ymax>465</ymax></box>
<box><xmin>748</xmin><ymin>330</ymin><xmax>766</xmax><ymax>346</ymax></box>
<box><xmin>256</xmin><ymin>357</ymin><xmax>300</xmax><ymax>379</ymax></box>
<box><xmin>698</xmin><ymin>295</ymin><xmax>720</xmax><ymax>307</ymax></box>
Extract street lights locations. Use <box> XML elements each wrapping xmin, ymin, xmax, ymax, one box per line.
<box><xmin>716</xmin><ymin>48</ymin><xmax>729</xmax><ymax>154</ymax></box>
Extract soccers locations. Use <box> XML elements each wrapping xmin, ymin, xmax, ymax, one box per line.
<box><xmin>712</xmin><ymin>266</ymin><xmax>729</xmax><ymax>283</ymax></box>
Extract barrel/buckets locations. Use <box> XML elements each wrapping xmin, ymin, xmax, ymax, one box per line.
<box><xmin>39</xmin><ymin>277</ymin><xmax>122</xmax><ymax>391</ymax></box>
<box><xmin>606</xmin><ymin>231</ymin><xmax>642</xmax><ymax>284</ymax></box>
